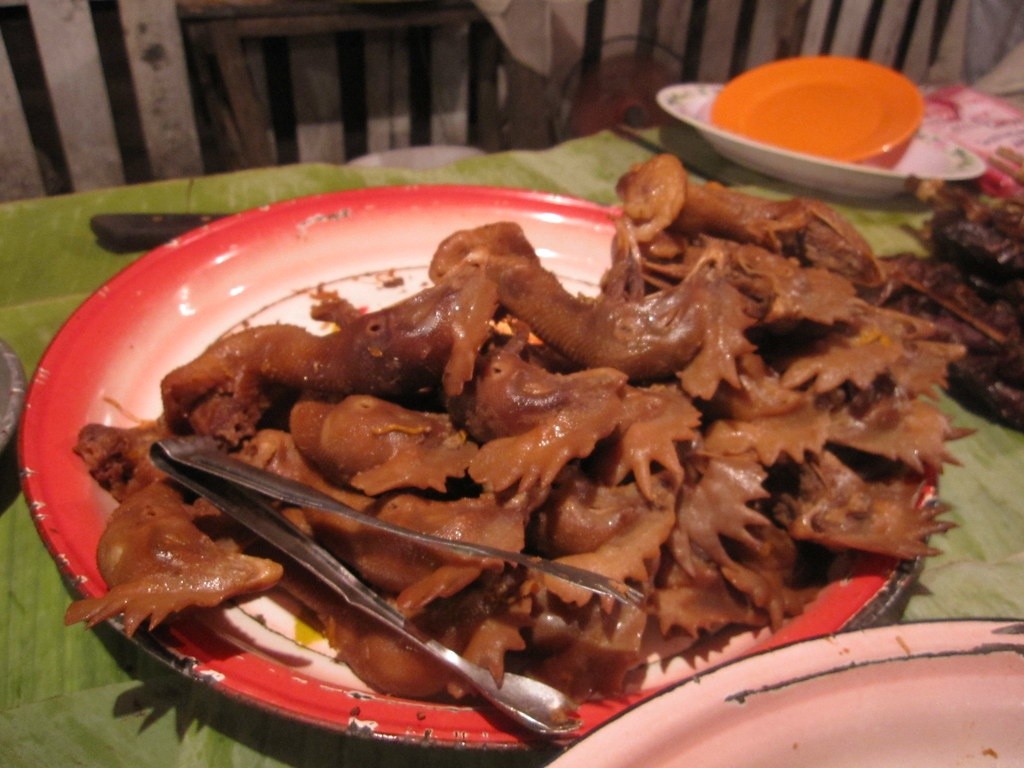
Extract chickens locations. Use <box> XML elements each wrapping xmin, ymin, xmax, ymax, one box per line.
<box><xmin>61</xmin><ymin>152</ymin><xmax>979</xmax><ymax>704</ymax></box>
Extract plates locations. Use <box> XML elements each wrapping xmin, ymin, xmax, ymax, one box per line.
<box><xmin>656</xmin><ymin>82</ymin><xmax>988</xmax><ymax>203</ymax></box>
<box><xmin>540</xmin><ymin>617</ymin><xmax>1024</xmax><ymax>768</ymax></box>
<box><xmin>20</xmin><ymin>185</ymin><xmax>941</xmax><ymax>753</ymax></box>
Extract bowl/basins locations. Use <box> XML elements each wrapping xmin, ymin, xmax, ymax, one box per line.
<box><xmin>711</xmin><ymin>56</ymin><xmax>925</xmax><ymax>164</ymax></box>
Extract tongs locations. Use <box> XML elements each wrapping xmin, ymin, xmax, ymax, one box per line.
<box><xmin>147</xmin><ymin>434</ymin><xmax>645</xmax><ymax>736</ymax></box>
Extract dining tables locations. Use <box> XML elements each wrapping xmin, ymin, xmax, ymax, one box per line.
<box><xmin>0</xmin><ymin>49</ymin><xmax>1024</xmax><ymax>768</ymax></box>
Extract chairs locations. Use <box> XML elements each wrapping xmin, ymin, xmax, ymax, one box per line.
<box><xmin>1</xmin><ymin>2</ymin><xmax>957</xmax><ymax>203</ymax></box>
